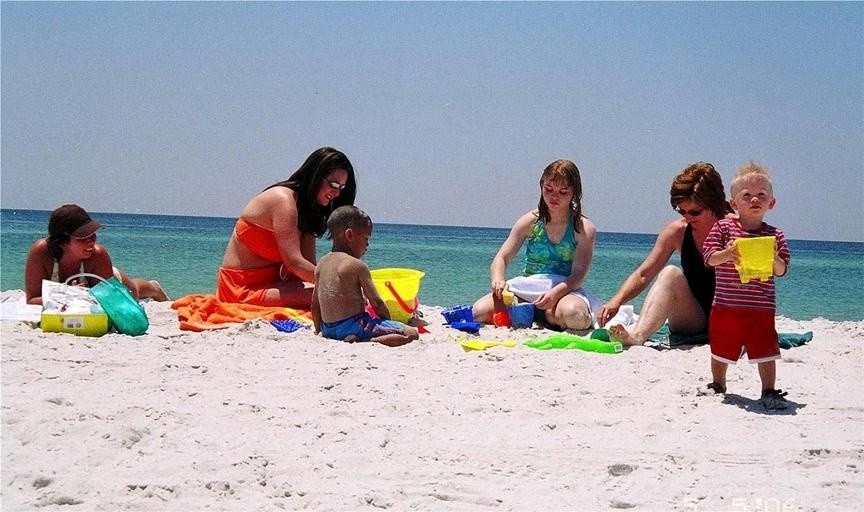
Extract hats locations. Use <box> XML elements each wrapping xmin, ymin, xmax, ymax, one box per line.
<box><xmin>47</xmin><ymin>204</ymin><xmax>107</xmax><ymax>240</ymax></box>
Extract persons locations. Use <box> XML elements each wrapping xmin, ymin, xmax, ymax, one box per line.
<box><xmin>26</xmin><ymin>204</ymin><xmax>168</xmax><ymax>304</ymax></box>
<box><xmin>312</xmin><ymin>206</ymin><xmax>419</xmax><ymax>346</ymax></box>
<box><xmin>472</xmin><ymin>160</ymin><xmax>595</xmax><ymax>330</ymax></box>
<box><xmin>703</xmin><ymin>160</ymin><xmax>790</xmax><ymax>410</ymax></box>
<box><xmin>216</xmin><ymin>147</ymin><xmax>357</xmax><ymax>312</ymax></box>
<box><xmin>597</xmin><ymin>161</ymin><xmax>735</xmax><ymax>347</ymax></box>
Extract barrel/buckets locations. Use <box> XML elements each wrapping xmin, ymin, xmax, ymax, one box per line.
<box><xmin>369</xmin><ymin>267</ymin><xmax>427</xmax><ymax>326</ymax></box>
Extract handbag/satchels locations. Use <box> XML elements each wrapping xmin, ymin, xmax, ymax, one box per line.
<box><xmin>39</xmin><ymin>277</ymin><xmax>149</xmax><ymax>337</ymax></box>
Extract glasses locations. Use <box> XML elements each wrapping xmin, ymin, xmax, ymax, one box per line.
<box><xmin>326</xmin><ymin>178</ymin><xmax>346</xmax><ymax>191</ymax></box>
<box><xmin>675</xmin><ymin>204</ymin><xmax>707</xmax><ymax>217</ymax></box>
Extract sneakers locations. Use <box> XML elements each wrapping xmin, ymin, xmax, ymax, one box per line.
<box><xmin>761</xmin><ymin>388</ymin><xmax>790</xmax><ymax>411</ymax></box>
<box><xmin>705</xmin><ymin>382</ymin><xmax>727</xmax><ymax>397</ymax></box>
